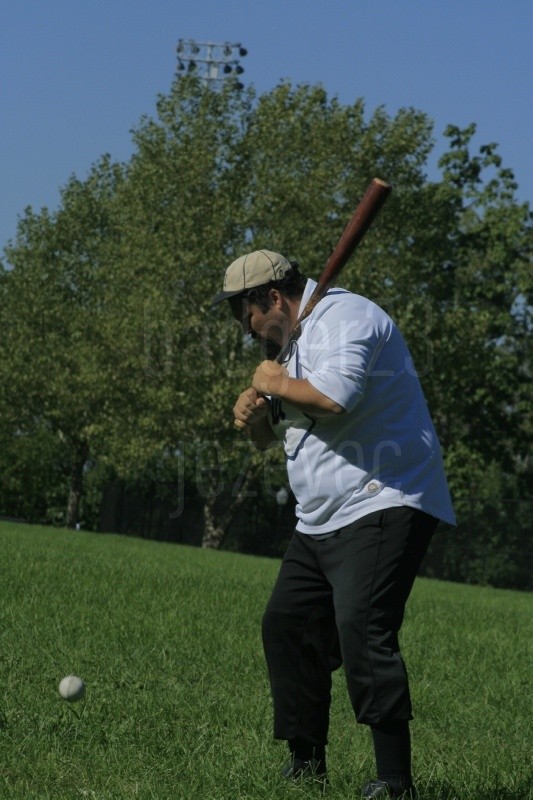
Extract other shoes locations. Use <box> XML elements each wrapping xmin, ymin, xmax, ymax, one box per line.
<box><xmin>353</xmin><ymin>777</ymin><xmax>417</xmax><ymax>799</ymax></box>
<box><xmin>268</xmin><ymin>766</ymin><xmax>329</xmax><ymax>791</ymax></box>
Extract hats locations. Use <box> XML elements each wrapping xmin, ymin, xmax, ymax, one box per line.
<box><xmin>212</xmin><ymin>249</ymin><xmax>292</xmax><ymax>305</ymax></box>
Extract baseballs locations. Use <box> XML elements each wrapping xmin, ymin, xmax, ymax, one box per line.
<box><xmin>59</xmin><ymin>676</ymin><xmax>85</xmax><ymax>701</ymax></box>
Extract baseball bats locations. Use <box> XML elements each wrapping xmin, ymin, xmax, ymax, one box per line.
<box><xmin>234</xmin><ymin>177</ymin><xmax>392</xmax><ymax>429</ymax></box>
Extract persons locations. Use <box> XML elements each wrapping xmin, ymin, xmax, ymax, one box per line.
<box><xmin>210</xmin><ymin>248</ymin><xmax>457</xmax><ymax>800</ymax></box>
